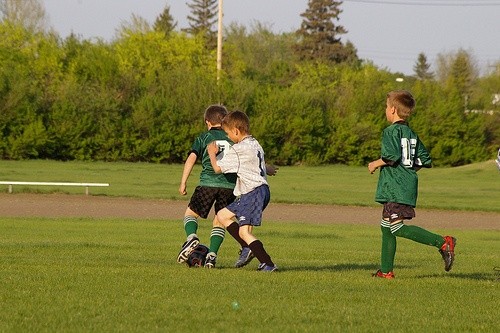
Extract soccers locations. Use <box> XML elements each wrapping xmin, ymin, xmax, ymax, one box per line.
<box><xmin>186</xmin><ymin>244</ymin><xmax>209</xmax><ymax>268</ymax></box>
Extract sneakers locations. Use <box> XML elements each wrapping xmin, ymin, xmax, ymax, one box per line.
<box><xmin>204</xmin><ymin>252</ymin><xmax>216</xmax><ymax>268</ymax></box>
<box><xmin>235</xmin><ymin>247</ymin><xmax>255</xmax><ymax>268</ymax></box>
<box><xmin>256</xmin><ymin>263</ymin><xmax>279</xmax><ymax>272</ymax></box>
<box><xmin>438</xmin><ymin>236</ymin><xmax>457</xmax><ymax>272</ymax></box>
<box><xmin>372</xmin><ymin>269</ymin><xmax>395</xmax><ymax>279</ymax></box>
<box><xmin>177</xmin><ymin>237</ymin><xmax>200</xmax><ymax>265</ymax></box>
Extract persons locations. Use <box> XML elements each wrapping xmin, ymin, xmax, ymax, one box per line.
<box><xmin>368</xmin><ymin>91</ymin><xmax>457</xmax><ymax>281</ymax></box>
<box><xmin>176</xmin><ymin>105</ymin><xmax>279</xmax><ymax>267</ymax></box>
<box><xmin>206</xmin><ymin>111</ymin><xmax>278</xmax><ymax>272</ymax></box>
